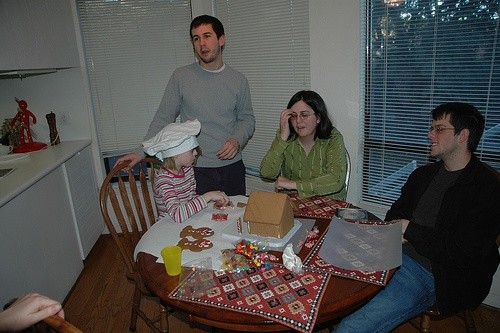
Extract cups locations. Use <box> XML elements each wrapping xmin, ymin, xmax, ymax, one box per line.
<box><xmin>161</xmin><ymin>246</ymin><xmax>182</xmax><ymax>276</ymax></box>
<box><xmin>0</xmin><ymin>145</ymin><xmax>13</xmax><ymax>155</ymax></box>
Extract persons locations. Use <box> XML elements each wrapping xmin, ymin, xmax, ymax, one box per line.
<box><xmin>260</xmin><ymin>90</ymin><xmax>347</xmax><ymax>202</ymax></box>
<box><xmin>142</xmin><ymin>119</ymin><xmax>230</xmax><ymax>223</ymax></box>
<box><xmin>114</xmin><ymin>15</ymin><xmax>256</xmax><ymax>196</ymax></box>
<box><xmin>335</xmin><ymin>102</ymin><xmax>500</xmax><ymax>333</ymax></box>
<box><xmin>10</xmin><ymin>100</ymin><xmax>36</xmax><ymax>145</ymax></box>
<box><xmin>0</xmin><ymin>291</ymin><xmax>65</xmax><ymax>333</ymax></box>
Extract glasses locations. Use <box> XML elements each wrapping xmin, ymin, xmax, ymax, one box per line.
<box><xmin>289</xmin><ymin>112</ymin><xmax>315</xmax><ymax>120</ymax></box>
<box><xmin>430</xmin><ymin>127</ymin><xmax>458</xmax><ymax>133</ymax></box>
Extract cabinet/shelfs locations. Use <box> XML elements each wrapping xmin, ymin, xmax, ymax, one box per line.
<box><xmin>0</xmin><ymin>0</ymin><xmax>80</xmax><ymax>78</ymax></box>
<box><xmin>61</xmin><ymin>145</ymin><xmax>105</xmax><ymax>261</ymax></box>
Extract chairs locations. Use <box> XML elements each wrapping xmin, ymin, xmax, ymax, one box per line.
<box><xmin>99</xmin><ymin>159</ymin><xmax>177</xmax><ymax>333</ymax></box>
<box><xmin>409</xmin><ymin>305</ymin><xmax>478</xmax><ymax>333</ymax></box>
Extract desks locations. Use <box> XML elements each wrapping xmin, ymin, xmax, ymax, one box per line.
<box><xmin>138</xmin><ymin>194</ymin><xmax>394</xmax><ymax>333</ymax></box>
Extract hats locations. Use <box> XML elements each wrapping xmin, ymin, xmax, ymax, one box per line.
<box><xmin>142</xmin><ymin>119</ymin><xmax>202</xmax><ymax>162</ymax></box>
